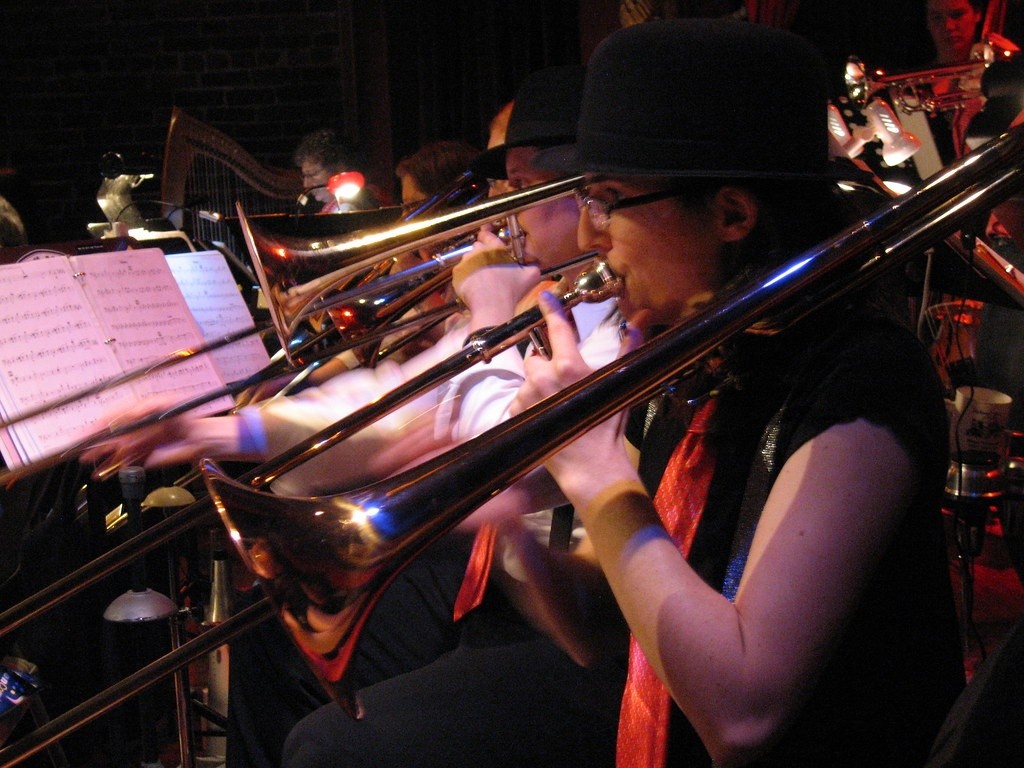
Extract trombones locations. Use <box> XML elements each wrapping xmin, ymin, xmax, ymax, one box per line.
<box><xmin>0</xmin><ymin>105</ymin><xmax>1024</xmax><ymax>768</ymax></box>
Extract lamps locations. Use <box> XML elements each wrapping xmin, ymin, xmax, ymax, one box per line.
<box><xmin>827</xmin><ymin>104</ymin><xmax>865</xmax><ymax>160</ymax></box>
<box><xmin>866</xmin><ymin>99</ymin><xmax>922</xmax><ymax>166</ymax></box>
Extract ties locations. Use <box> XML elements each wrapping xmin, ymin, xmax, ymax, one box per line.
<box><xmin>453</xmin><ymin>522</ymin><xmax>501</xmax><ymax>625</ymax></box>
<box><xmin>613</xmin><ymin>396</ymin><xmax>718</xmax><ymax>768</ymax></box>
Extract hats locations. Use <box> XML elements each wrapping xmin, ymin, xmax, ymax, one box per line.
<box><xmin>474</xmin><ymin>101</ymin><xmax>516</xmax><ymax>186</ymax></box>
<box><xmin>570</xmin><ymin>17</ymin><xmax>838</xmax><ymax>181</ymax></box>
<box><xmin>469</xmin><ymin>63</ymin><xmax>587</xmax><ymax>187</ymax></box>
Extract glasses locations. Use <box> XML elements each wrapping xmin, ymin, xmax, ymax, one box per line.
<box><xmin>399</xmin><ymin>200</ymin><xmax>425</xmax><ymax>211</ymax></box>
<box><xmin>571</xmin><ymin>183</ymin><xmax>712</xmax><ymax>230</ymax></box>
<box><xmin>299</xmin><ymin>166</ymin><xmax>325</xmax><ymax>181</ymax></box>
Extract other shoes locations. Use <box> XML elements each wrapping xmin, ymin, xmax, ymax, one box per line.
<box><xmin>0</xmin><ymin>666</ymin><xmax>45</xmax><ymax>717</ymax></box>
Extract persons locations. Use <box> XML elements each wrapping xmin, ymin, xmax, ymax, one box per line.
<box><xmin>0</xmin><ymin>168</ymin><xmax>188</xmax><ymax>767</ymax></box>
<box><xmin>820</xmin><ymin>0</ymin><xmax>1024</xmax><ymax>372</ymax></box>
<box><xmin>287</xmin><ymin>16</ymin><xmax>951</xmax><ymax>768</ymax></box>
<box><xmin>211</xmin><ymin>84</ymin><xmax>639</xmax><ymax>460</ymax></box>
